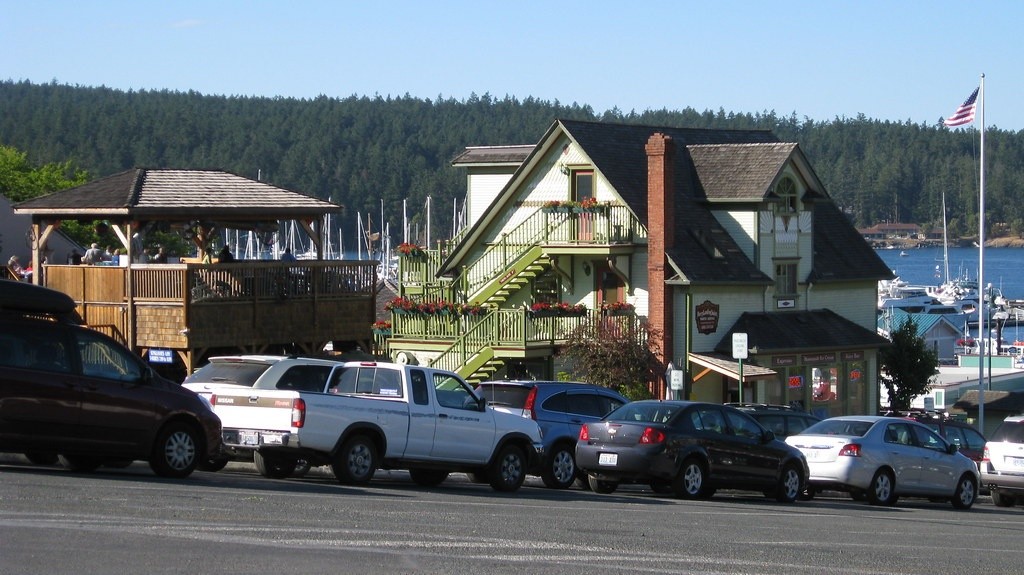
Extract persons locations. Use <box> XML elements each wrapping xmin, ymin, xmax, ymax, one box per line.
<box><xmin>219</xmin><ymin>246</ymin><xmax>235</xmax><ymax>263</ymax></box>
<box><xmin>282</xmin><ymin>249</ymin><xmax>296</xmax><ymax>262</ymax></box>
<box><xmin>201</xmin><ymin>247</ymin><xmax>212</xmax><ymax>264</ymax></box>
<box><xmin>138</xmin><ymin>246</ymin><xmax>150</xmax><ymax>264</ymax></box>
<box><xmin>81</xmin><ymin>243</ymin><xmax>119</xmax><ymax>266</ymax></box>
<box><xmin>8</xmin><ymin>255</ymin><xmax>32</xmax><ymax>278</ymax></box>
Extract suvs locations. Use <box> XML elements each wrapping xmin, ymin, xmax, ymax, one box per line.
<box><xmin>0</xmin><ymin>279</ymin><xmax>224</xmax><ymax>479</ymax></box>
<box><xmin>894</xmin><ymin>413</ymin><xmax>989</xmax><ymax>470</ymax></box>
<box><xmin>979</xmin><ymin>415</ymin><xmax>1024</xmax><ymax>507</ymax></box>
<box><xmin>181</xmin><ymin>354</ymin><xmax>360</xmax><ymax>473</ymax></box>
<box><xmin>463</xmin><ymin>380</ymin><xmax>632</xmax><ymax>490</ymax></box>
<box><xmin>692</xmin><ymin>402</ymin><xmax>833</xmax><ymax>501</ymax></box>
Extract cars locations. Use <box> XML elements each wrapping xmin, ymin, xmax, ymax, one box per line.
<box><xmin>573</xmin><ymin>400</ymin><xmax>810</xmax><ymax>502</ymax></box>
<box><xmin>784</xmin><ymin>415</ymin><xmax>979</xmax><ymax>511</ymax></box>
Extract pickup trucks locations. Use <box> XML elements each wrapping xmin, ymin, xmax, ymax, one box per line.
<box><xmin>206</xmin><ymin>351</ymin><xmax>546</xmax><ymax>492</ymax></box>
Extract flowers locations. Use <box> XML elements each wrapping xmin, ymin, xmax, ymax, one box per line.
<box><xmin>371</xmin><ymin>321</ymin><xmax>392</xmax><ymax>334</ymax></box>
<box><xmin>601</xmin><ymin>302</ymin><xmax>634</xmax><ymax>316</ymax></box>
<box><xmin>542</xmin><ymin>197</ymin><xmax>607</xmax><ymax>212</ymax></box>
<box><xmin>532</xmin><ymin>300</ymin><xmax>587</xmax><ymax>316</ymax></box>
<box><xmin>393</xmin><ymin>242</ymin><xmax>424</xmax><ymax>260</ymax></box>
<box><xmin>384</xmin><ymin>296</ymin><xmax>482</xmax><ymax>316</ymax></box>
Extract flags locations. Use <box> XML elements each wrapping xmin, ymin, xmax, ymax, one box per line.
<box><xmin>945</xmin><ymin>81</ymin><xmax>981</xmax><ymax>127</ymax></box>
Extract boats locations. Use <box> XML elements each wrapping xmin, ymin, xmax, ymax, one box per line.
<box><xmin>878</xmin><ymin>190</ymin><xmax>1024</xmax><ymax>331</ymax></box>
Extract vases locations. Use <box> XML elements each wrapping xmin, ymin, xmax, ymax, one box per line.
<box><xmin>392</xmin><ymin>307</ymin><xmax>486</xmax><ymax>316</ymax></box>
<box><xmin>373</xmin><ymin>329</ymin><xmax>391</xmax><ymax>335</ymax></box>
<box><xmin>535</xmin><ymin>310</ymin><xmax>583</xmax><ymax>317</ymax></box>
<box><xmin>543</xmin><ymin>207</ymin><xmax>605</xmax><ymax>213</ymax></box>
<box><xmin>603</xmin><ymin>310</ymin><xmax>633</xmax><ymax>316</ymax></box>
<box><xmin>397</xmin><ymin>251</ymin><xmax>423</xmax><ymax>257</ymax></box>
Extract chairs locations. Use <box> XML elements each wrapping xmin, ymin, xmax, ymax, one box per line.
<box><xmin>886</xmin><ymin>430</ymin><xmax>904</xmax><ymax>444</ymax></box>
<box><xmin>0</xmin><ymin>341</ymin><xmax>16</xmax><ymax>366</ymax></box>
<box><xmin>853</xmin><ymin>424</ymin><xmax>869</xmax><ymax>435</ymax></box>
<box><xmin>30</xmin><ymin>346</ymin><xmax>67</xmax><ymax>372</ymax></box>
<box><xmin>701</xmin><ymin>414</ymin><xmax>720</xmax><ymax>434</ymax></box>
